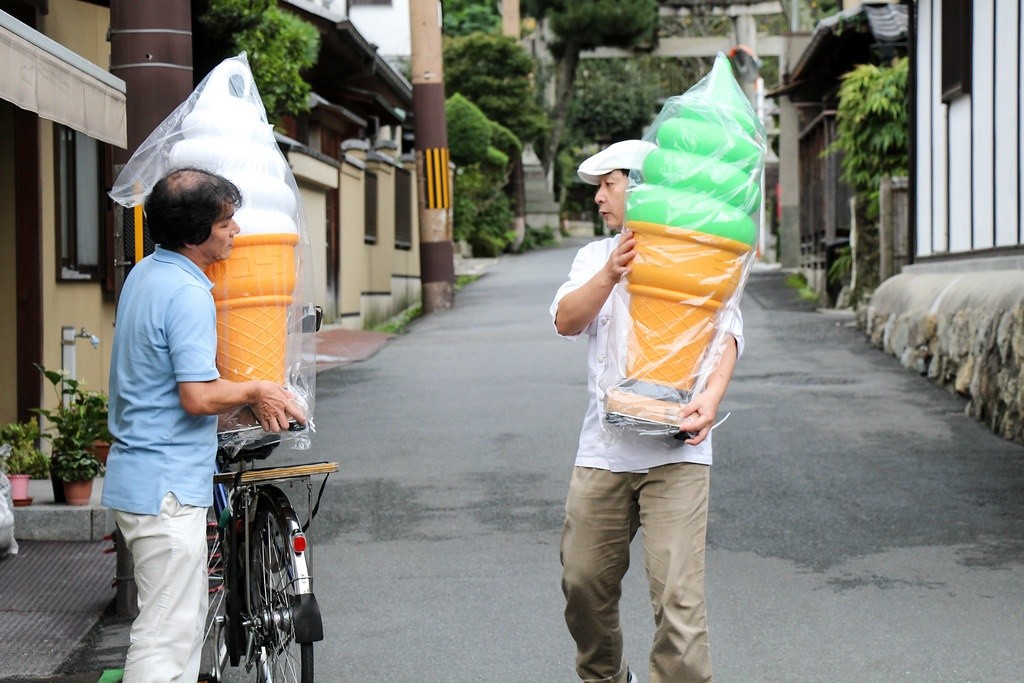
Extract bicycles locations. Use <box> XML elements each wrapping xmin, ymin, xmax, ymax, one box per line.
<box><xmin>211</xmin><ymin>415</ymin><xmax>339</xmax><ymax>683</ymax></box>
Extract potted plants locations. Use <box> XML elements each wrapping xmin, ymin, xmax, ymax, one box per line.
<box><xmin>27</xmin><ymin>362</ymin><xmax>108</xmax><ymax>507</ymax></box>
<box><xmin>87</xmin><ymin>394</ymin><xmax>111</xmax><ymax>468</ymax></box>
<box><xmin>0</xmin><ymin>416</ymin><xmax>53</xmax><ymax>499</ymax></box>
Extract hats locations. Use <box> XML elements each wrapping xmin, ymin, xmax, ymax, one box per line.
<box><xmin>577</xmin><ymin>139</ymin><xmax>659</xmax><ymax>186</ymax></box>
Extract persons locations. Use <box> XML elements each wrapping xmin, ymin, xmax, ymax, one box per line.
<box><xmin>548</xmin><ymin>139</ymin><xmax>745</xmax><ymax>683</ymax></box>
<box><xmin>100</xmin><ymin>164</ymin><xmax>307</xmax><ymax>683</ymax></box>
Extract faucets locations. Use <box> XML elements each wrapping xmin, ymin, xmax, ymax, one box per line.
<box><xmin>74</xmin><ymin>323</ymin><xmax>103</xmax><ymax>350</ymax></box>
